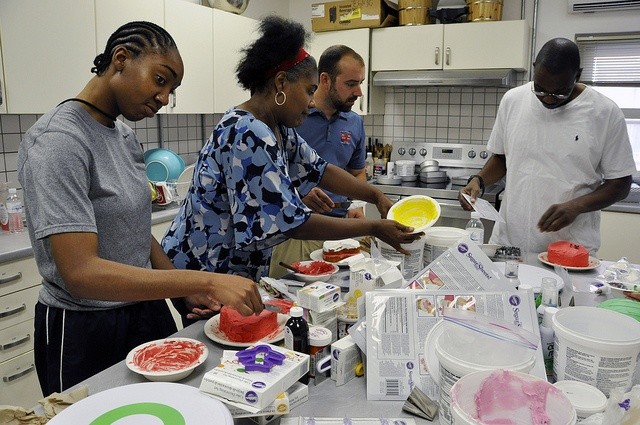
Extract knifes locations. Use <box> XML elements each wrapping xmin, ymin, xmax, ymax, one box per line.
<box><xmin>327</xmin><ymin>201</ymin><xmax>356</xmax><ymax>209</ymax></box>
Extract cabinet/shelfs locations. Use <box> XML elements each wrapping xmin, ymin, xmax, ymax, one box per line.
<box><xmin>213</xmin><ymin>7</ymin><xmax>264</xmax><ymax>114</ymax></box>
<box><xmin>2</xmin><ymin>0</ymin><xmax>96</xmax><ymax>115</ymax></box>
<box><xmin>94</xmin><ymin>2</ymin><xmax>211</xmax><ymax>115</ymax></box>
<box><xmin>0</xmin><ymin>256</ymin><xmax>42</xmax><ymax>388</ymax></box>
<box><xmin>369</xmin><ymin>20</ymin><xmax>532</xmax><ymax>73</ymax></box>
<box><xmin>308</xmin><ymin>28</ymin><xmax>386</xmax><ymax>115</ymax></box>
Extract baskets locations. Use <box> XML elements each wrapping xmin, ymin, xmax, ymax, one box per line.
<box><xmin>166</xmin><ymin>183</ymin><xmax>190</xmax><ymax>201</ymax></box>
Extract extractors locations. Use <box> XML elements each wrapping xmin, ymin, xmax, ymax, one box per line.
<box><xmin>371</xmin><ymin>70</ymin><xmax>519</xmax><ymax>90</ymax></box>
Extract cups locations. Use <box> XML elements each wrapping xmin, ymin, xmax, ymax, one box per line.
<box><xmin>154</xmin><ymin>181</ymin><xmax>173</xmax><ymax>207</ymax></box>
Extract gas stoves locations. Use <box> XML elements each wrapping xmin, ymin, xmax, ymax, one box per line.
<box><xmin>366</xmin><ymin>139</ymin><xmax>504</xmax><ymax>202</ymax></box>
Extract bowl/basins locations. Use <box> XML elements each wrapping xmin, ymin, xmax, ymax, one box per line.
<box><xmin>143</xmin><ymin>146</ymin><xmax>185</xmax><ymax>185</ymax></box>
<box><xmin>125</xmin><ymin>339</ymin><xmax>207</xmax><ymax>381</ymax></box>
<box><xmin>476</xmin><ymin>244</ymin><xmax>502</xmax><ymax>259</ymax></box>
<box><xmin>285</xmin><ymin>261</ymin><xmax>340</xmax><ymax>282</ymax></box>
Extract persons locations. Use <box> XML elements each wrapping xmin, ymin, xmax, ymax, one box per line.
<box><xmin>160</xmin><ymin>13</ymin><xmax>424</xmax><ymax>329</ymax></box>
<box><xmin>458</xmin><ymin>36</ymin><xmax>638</xmax><ymax>258</ymax></box>
<box><xmin>17</xmin><ymin>20</ymin><xmax>265</xmax><ymax>398</ymax></box>
<box><xmin>269</xmin><ymin>44</ymin><xmax>367</xmax><ymax>281</ymax></box>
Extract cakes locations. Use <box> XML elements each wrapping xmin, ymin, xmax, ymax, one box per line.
<box><xmin>218</xmin><ymin>308</ymin><xmax>278</xmax><ymax>341</ymax></box>
<box><xmin>548</xmin><ymin>242</ymin><xmax>592</xmax><ymax>267</ymax></box>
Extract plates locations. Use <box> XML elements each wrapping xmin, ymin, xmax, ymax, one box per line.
<box><xmin>42</xmin><ymin>382</ymin><xmax>236</xmax><ymax>425</ymax></box>
<box><xmin>538</xmin><ymin>251</ymin><xmax>602</xmax><ymax>270</ymax></box>
<box><xmin>309</xmin><ymin>247</ymin><xmax>371</xmax><ymax>267</ymax></box>
<box><xmin>176</xmin><ymin>162</ymin><xmax>196</xmax><ymax>201</ymax></box>
<box><xmin>386</xmin><ymin>196</ymin><xmax>441</xmax><ymax>234</ymax></box>
<box><xmin>204</xmin><ymin>314</ymin><xmax>288</xmax><ymax>348</ymax></box>
<box><xmin>484</xmin><ymin>262</ymin><xmax>565</xmax><ymax>296</ymax></box>
<box><xmin>398</xmin><ymin>173</ymin><xmax>418</xmax><ymax>182</ymax></box>
<box><xmin>418</xmin><ymin>158</ymin><xmax>450</xmax><ymax>184</ymax></box>
<box><xmin>375</xmin><ymin>175</ymin><xmax>402</xmax><ymax>185</ymax></box>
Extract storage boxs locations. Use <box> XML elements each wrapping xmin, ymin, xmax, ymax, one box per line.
<box><xmin>310</xmin><ymin>0</ymin><xmax>398</xmax><ymax>33</ymax></box>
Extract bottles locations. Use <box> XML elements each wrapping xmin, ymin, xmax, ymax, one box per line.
<box><xmin>304</xmin><ymin>327</ymin><xmax>331</xmax><ymax>378</ymax></box>
<box><xmin>505</xmin><ymin>261</ymin><xmax>520</xmax><ymax>286</ymax></box>
<box><xmin>285</xmin><ymin>306</ymin><xmax>307</xmax><ymax>384</ymax></box>
<box><xmin>0</xmin><ymin>195</ymin><xmax>10</xmax><ymax>231</ymax></box>
<box><xmin>466</xmin><ymin>213</ymin><xmax>483</xmax><ymax>245</ymax></box>
<box><xmin>366</xmin><ymin>152</ymin><xmax>373</xmax><ymax>177</ymax></box>
<box><xmin>374</xmin><ymin>166</ymin><xmax>382</xmax><ymax>175</ymax></box>
<box><xmin>540</xmin><ymin>308</ymin><xmax>555</xmax><ymax>376</ymax></box>
<box><xmin>7</xmin><ymin>187</ymin><xmax>24</xmax><ymax>234</ymax></box>
<box><xmin>538</xmin><ymin>279</ymin><xmax>561</xmax><ymax>316</ymax></box>
<box><xmin>387</xmin><ymin>162</ymin><xmax>394</xmax><ymax>175</ymax></box>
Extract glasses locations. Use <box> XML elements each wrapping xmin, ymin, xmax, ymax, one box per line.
<box><xmin>531</xmin><ymin>72</ymin><xmax>579</xmax><ymax>100</ymax></box>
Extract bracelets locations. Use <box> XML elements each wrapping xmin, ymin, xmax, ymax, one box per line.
<box><xmin>467</xmin><ymin>175</ymin><xmax>485</xmax><ymax>198</ymax></box>
